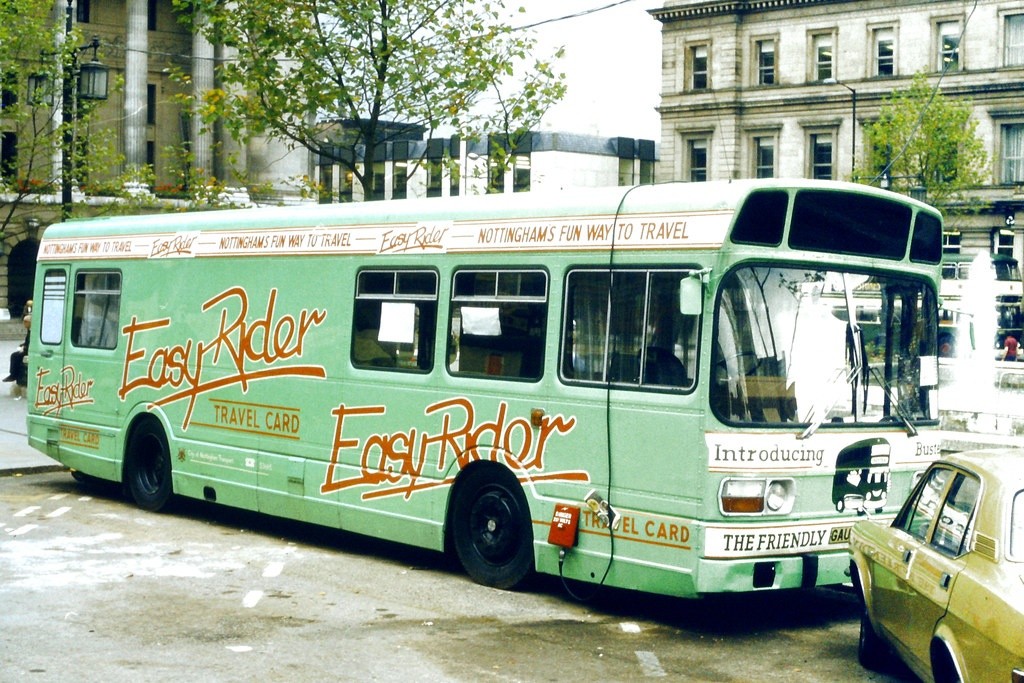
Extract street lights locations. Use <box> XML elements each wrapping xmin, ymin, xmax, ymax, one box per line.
<box><xmin>823</xmin><ymin>77</ymin><xmax>858</xmax><ymax>183</ymax></box>
<box><xmin>467</xmin><ymin>151</ymin><xmax>491</xmax><ymax>187</ymax></box>
<box><xmin>26</xmin><ymin>0</ymin><xmax>113</xmax><ymax>222</ymax></box>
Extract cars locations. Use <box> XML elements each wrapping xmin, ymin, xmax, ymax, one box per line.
<box><xmin>845</xmin><ymin>446</ymin><xmax>1024</xmax><ymax>683</ymax></box>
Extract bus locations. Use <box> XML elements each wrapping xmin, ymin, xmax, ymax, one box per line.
<box><xmin>24</xmin><ymin>174</ymin><xmax>978</xmax><ymax>603</ymax></box>
<box><xmin>742</xmin><ymin>244</ymin><xmax>1022</xmax><ymax>408</ymax></box>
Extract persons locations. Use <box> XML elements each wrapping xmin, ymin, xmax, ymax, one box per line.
<box><xmin>2</xmin><ymin>300</ymin><xmax>33</xmax><ymax>387</ymax></box>
<box><xmin>1001</xmin><ymin>331</ymin><xmax>1018</xmax><ymax>361</ymax></box>
<box><xmin>938</xmin><ymin>340</ymin><xmax>951</xmax><ymax>357</ymax></box>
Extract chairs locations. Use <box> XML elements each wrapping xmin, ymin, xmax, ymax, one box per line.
<box><xmin>637</xmin><ymin>346</ymin><xmax>690</xmax><ymax>388</ymax></box>
